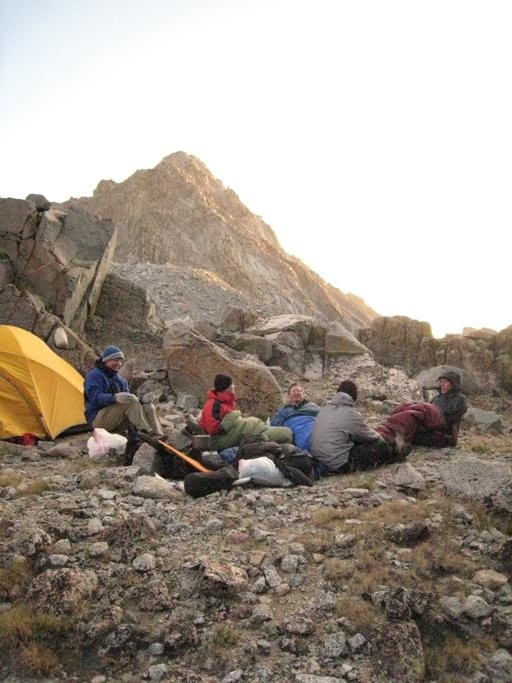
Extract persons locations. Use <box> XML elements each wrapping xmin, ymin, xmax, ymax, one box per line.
<box><xmin>431</xmin><ymin>369</ymin><xmax>468</xmax><ymax>448</ymax></box>
<box><xmin>270</xmin><ymin>383</ymin><xmax>322</xmax><ymax>426</ymax></box>
<box><xmin>199</xmin><ymin>374</ymin><xmax>237</xmax><ymax>436</ymax></box>
<box><xmin>311</xmin><ymin>380</ymin><xmax>413</xmax><ymax>474</ymax></box>
<box><xmin>83</xmin><ymin>345</ymin><xmax>168</xmax><ymax>441</ymax></box>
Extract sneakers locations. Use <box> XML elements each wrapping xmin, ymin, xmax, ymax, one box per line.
<box><xmin>137</xmin><ymin>431</ymin><xmax>169</xmax><ymax>444</ymax></box>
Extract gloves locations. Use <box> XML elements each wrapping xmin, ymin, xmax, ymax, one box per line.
<box><xmin>114</xmin><ymin>392</ymin><xmax>138</xmax><ymax>404</ymax></box>
<box><xmin>141</xmin><ymin>391</ymin><xmax>157</xmax><ymax>404</ymax></box>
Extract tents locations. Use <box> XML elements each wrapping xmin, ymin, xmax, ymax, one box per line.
<box><xmin>0</xmin><ymin>324</ymin><xmax>88</xmax><ymax>442</ymax></box>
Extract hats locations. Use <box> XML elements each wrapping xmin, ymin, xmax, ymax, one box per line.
<box><xmin>101</xmin><ymin>344</ymin><xmax>125</xmax><ymax>362</ymax></box>
<box><xmin>338</xmin><ymin>380</ymin><xmax>358</xmax><ymax>401</ymax></box>
<box><xmin>214</xmin><ymin>374</ymin><xmax>232</xmax><ymax>392</ymax></box>
